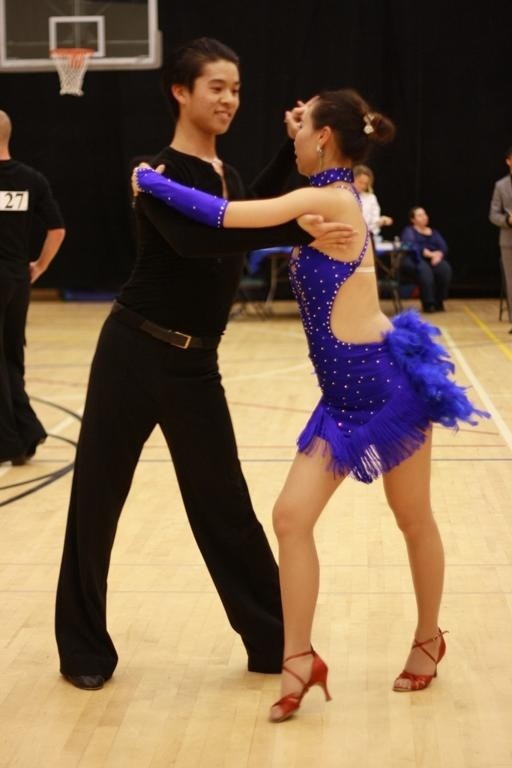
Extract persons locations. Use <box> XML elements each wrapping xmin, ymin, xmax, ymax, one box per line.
<box><xmin>52</xmin><ymin>34</ymin><xmax>361</xmax><ymax>690</ymax></box>
<box><xmin>1</xmin><ymin>105</ymin><xmax>69</xmax><ymax>470</ymax></box>
<box><xmin>486</xmin><ymin>146</ymin><xmax>511</xmax><ymax>335</ymax></box>
<box><xmin>399</xmin><ymin>205</ymin><xmax>453</xmax><ymax>313</ymax></box>
<box><xmin>129</xmin><ymin>87</ymin><xmax>496</xmax><ymax>723</ymax></box>
<box><xmin>350</xmin><ymin>164</ymin><xmax>393</xmax><ymax>246</ymax></box>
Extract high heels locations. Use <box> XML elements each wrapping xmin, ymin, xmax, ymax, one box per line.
<box><xmin>265</xmin><ymin>641</ymin><xmax>334</xmax><ymax>725</ymax></box>
<box><xmin>391</xmin><ymin>624</ymin><xmax>450</xmax><ymax>695</ymax></box>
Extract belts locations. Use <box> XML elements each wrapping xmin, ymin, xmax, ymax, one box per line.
<box><xmin>110</xmin><ymin>298</ymin><xmax>223</xmax><ymax>355</ymax></box>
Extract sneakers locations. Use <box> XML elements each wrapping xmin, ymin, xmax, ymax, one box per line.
<box><xmin>421</xmin><ymin>299</ymin><xmax>447</xmax><ymax>313</ymax></box>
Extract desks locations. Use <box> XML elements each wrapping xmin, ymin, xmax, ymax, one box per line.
<box><xmin>254</xmin><ymin>242</ymin><xmax>410</xmax><ymax>315</ymax></box>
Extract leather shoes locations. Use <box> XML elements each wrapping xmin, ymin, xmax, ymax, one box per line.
<box><xmin>10</xmin><ymin>431</ymin><xmax>48</xmax><ymax>468</ymax></box>
<box><xmin>63</xmin><ymin>673</ymin><xmax>108</xmax><ymax>692</ymax></box>
